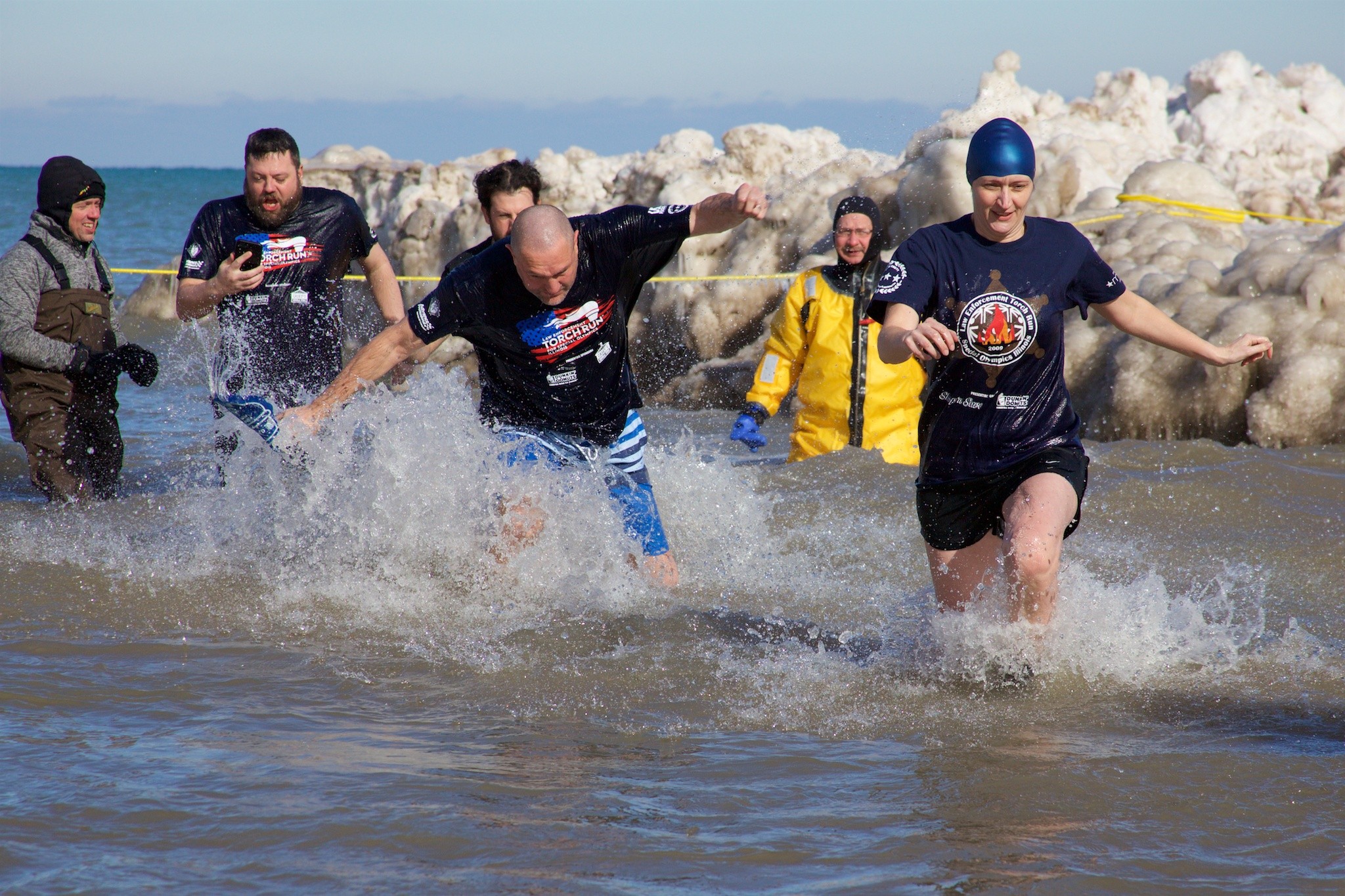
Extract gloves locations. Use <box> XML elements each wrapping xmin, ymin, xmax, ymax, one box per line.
<box><xmin>730</xmin><ymin>405</ymin><xmax>766</xmax><ymax>451</ymax></box>
<box><xmin>61</xmin><ymin>346</ymin><xmax>121</xmax><ymax>395</ymax></box>
<box><xmin>116</xmin><ymin>343</ymin><xmax>158</xmax><ymax>388</ymax></box>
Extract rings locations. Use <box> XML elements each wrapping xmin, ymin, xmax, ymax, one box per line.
<box><xmin>753</xmin><ymin>205</ymin><xmax>761</xmax><ymax>209</ymax></box>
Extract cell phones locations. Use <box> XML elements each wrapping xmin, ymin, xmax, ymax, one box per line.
<box><xmin>233</xmin><ymin>238</ymin><xmax>262</xmax><ymax>271</ymax></box>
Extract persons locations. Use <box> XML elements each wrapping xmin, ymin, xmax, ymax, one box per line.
<box><xmin>863</xmin><ymin>120</ymin><xmax>1272</xmax><ymax>629</ymax></box>
<box><xmin>0</xmin><ymin>155</ymin><xmax>161</xmax><ymax>507</ymax></box>
<box><xmin>274</xmin><ymin>183</ymin><xmax>774</xmax><ymax>582</ymax></box>
<box><xmin>174</xmin><ymin>128</ymin><xmax>414</xmax><ymax>489</ymax></box>
<box><xmin>391</xmin><ymin>158</ymin><xmax>545</xmax><ymax>382</ymax></box>
<box><xmin>728</xmin><ymin>195</ymin><xmax>924</xmax><ymax>466</ymax></box>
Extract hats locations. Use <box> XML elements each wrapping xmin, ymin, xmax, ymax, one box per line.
<box><xmin>37</xmin><ymin>156</ymin><xmax>106</xmax><ymax>246</ymax></box>
<box><xmin>966</xmin><ymin>119</ymin><xmax>1036</xmax><ymax>186</ymax></box>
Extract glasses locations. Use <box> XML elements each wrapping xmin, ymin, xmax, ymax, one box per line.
<box><xmin>834</xmin><ymin>230</ymin><xmax>872</xmax><ymax>237</ymax></box>
